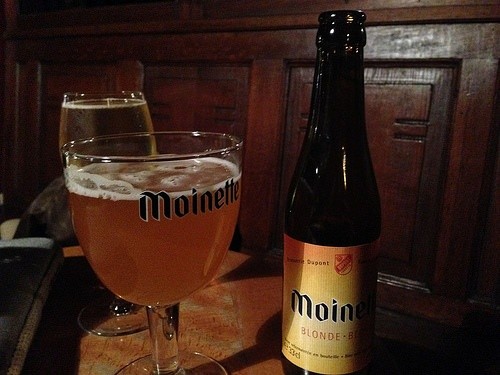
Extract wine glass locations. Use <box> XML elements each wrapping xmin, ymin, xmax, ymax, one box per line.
<box><xmin>61</xmin><ymin>131</ymin><xmax>244</xmax><ymax>375</ymax></box>
<box><xmin>58</xmin><ymin>91</ymin><xmax>158</xmax><ymax>337</ymax></box>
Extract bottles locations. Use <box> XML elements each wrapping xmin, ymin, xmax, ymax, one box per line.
<box><xmin>281</xmin><ymin>10</ymin><xmax>381</xmax><ymax>375</ymax></box>
